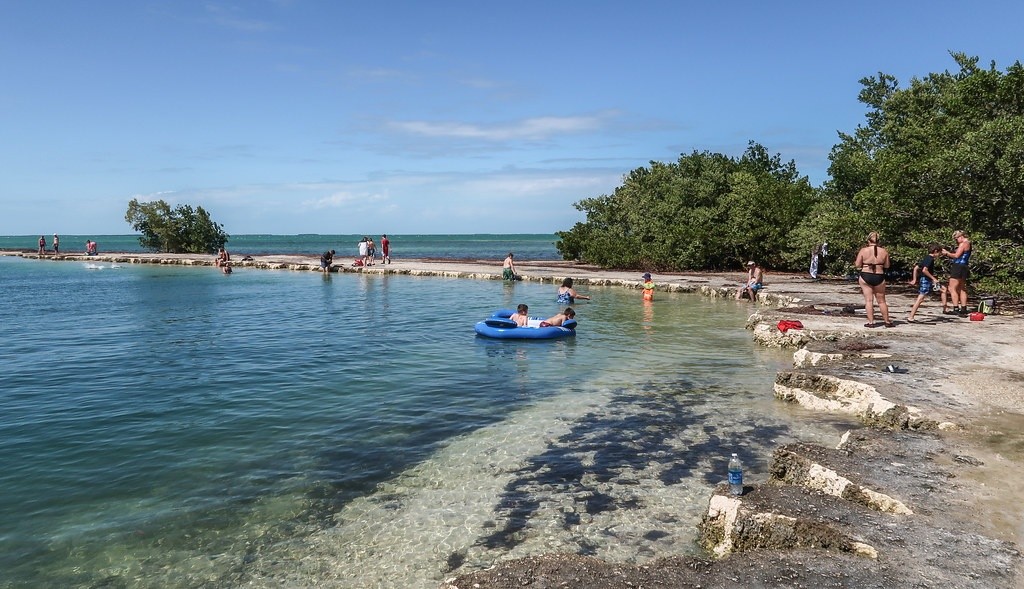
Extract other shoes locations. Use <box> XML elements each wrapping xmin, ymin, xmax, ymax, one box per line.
<box><xmin>908</xmin><ymin>319</ymin><xmax>920</xmax><ymax>323</ymax></box>
<box><xmin>943</xmin><ymin>309</ymin><xmax>952</xmax><ymax>316</ymax></box>
<box><xmin>886</xmin><ymin>322</ymin><xmax>895</xmax><ymax>326</ymax></box>
<box><xmin>952</xmin><ymin>309</ymin><xmax>967</xmax><ymax>318</ymax></box>
<box><xmin>864</xmin><ymin>323</ymin><xmax>875</xmax><ymax>328</ymax></box>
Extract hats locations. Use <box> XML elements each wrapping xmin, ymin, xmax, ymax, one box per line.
<box><xmin>747</xmin><ymin>261</ymin><xmax>755</xmax><ymax>266</ymax></box>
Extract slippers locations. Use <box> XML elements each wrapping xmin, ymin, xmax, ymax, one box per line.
<box><xmin>882</xmin><ymin>364</ymin><xmax>908</xmax><ymax>373</ymax></box>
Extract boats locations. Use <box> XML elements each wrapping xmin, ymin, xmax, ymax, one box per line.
<box><xmin>474</xmin><ymin>308</ymin><xmax>577</xmax><ymax>339</ymax></box>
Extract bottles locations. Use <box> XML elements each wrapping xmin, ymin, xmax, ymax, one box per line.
<box><xmin>727</xmin><ymin>453</ymin><xmax>743</xmax><ymax>495</ymax></box>
<box><xmin>970</xmin><ymin>312</ymin><xmax>985</xmax><ymax>321</ymax></box>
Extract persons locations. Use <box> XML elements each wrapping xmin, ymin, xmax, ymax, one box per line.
<box><xmin>503</xmin><ymin>253</ymin><xmax>518</xmax><ymax>281</ymax></box>
<box><xmin>738</xmin><ymin>260</ymin><xmax>762</xmax><ymax>303</ymax></box>
<box><xmin>544</xmin><ymin>307</ymin><xmax>576</xmax><ymax>326</ymax></box>
<box><xmin>942</xmin><ymin>229</ymin><xmax>971</xmax><ymax>315</ymax></box>
<box><xmin>320</xmin><ymin>250</ymin><xmax>336</xmax><ymax>272</ymax></box>
<box><xmin>358</xmin><ymin>237</ymin><xmax>377</xmax><ymax>266</ymax></box>
<box><xmin>38</xmin><ymin>236</ymin><xmax>47</xmax><ymax>255</ymax></box>
<box><xmin>509</xmin><ymin>304</ymin><xmax>529</xmax><ymax>327</ymax></box>
<box><xmin>855</xmin><ymin>231</ymin><xmax>895</xmax><ymax>328</ymax></box>
<box><xmin>53</xmin><ymin>233</ymin><xmax>60</xmax><ymax>255</ymax></box>
<box><xmin>214</xmin><ymin>248</ymin><xmax>229</xmax><ymax>267</ymax></box>
<box><xmin>557</xmin><ymin>278</ymin><xmax>590</xmax><ymax>304</ymax></box>
<box><xmin>638</xmin><ymin>273</ymin><xmax>656</xmax><ymax>300</ymax></box>
<box><xmin>381</xmin><ymin>234</ymin><xmax>390</xmax><ymax>264</ymax></box>
<box><xmin>223</xmin><ymin>263</ymin><xmax>232</xmax><ymax>274</ymax></box>
<box><xmin>906</xmin><ymin>243</ymin><xmax>948</xmax><ymax>323</ymax></box>
<box><xmin>86</xmin><ymin>240</ymin><xmax>98</xmax><ymax>256</ymax></box>
